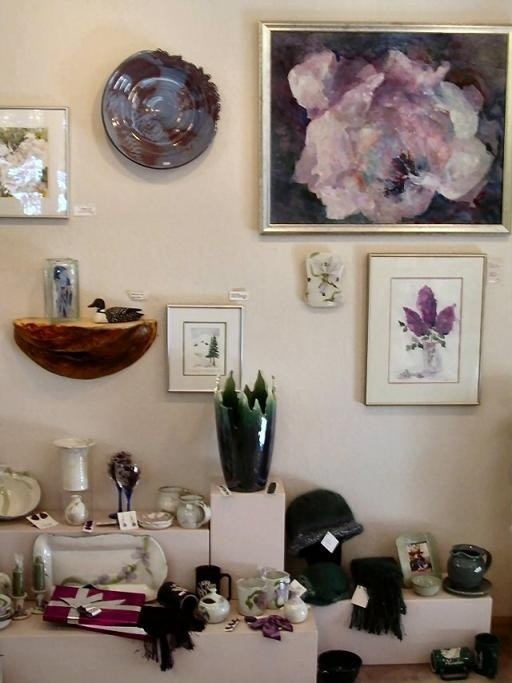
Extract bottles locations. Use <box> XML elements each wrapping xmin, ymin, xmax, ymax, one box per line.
<box><xmin>61</xmin><ymin>492</ymin><xmax>91</xmax><ymax>527</ymax></box>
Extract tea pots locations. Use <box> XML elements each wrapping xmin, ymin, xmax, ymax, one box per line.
<box><xmin>446</xmin><ymin>544</ymin><xmax>492</xmax><ymax>587</ymax></box>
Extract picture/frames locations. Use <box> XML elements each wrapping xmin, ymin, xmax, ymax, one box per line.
<box><xmin>0</xmin><ymin>106</ymin><xmax>70</xmax><ymax>219</ymax></box>
<box><xmin>395</xmin><ymin>532</ymin><xmax>442</xmax><ymax>587</ymax></box>
<box><xmin>166</xmin><ymin>305</ymin><xmax>243</xmax><ymax>393</ymax></box>
<box><xmin>258</xmin><ymin>19</ymin><xmax>512</xmax><ymax>234</ymax></box>
<box><xmin>363</xmin><ymin>253</ymin><xmax>487</xmax><ymax>406</ymax></box>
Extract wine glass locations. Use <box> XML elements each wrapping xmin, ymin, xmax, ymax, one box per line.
<box><xmin>106</xmin><ymin>453</ymin><xmax>141</xmax><ymax>525</ymax></box>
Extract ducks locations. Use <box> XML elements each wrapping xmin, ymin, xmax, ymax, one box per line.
<box><xmin>88</xmin><ymin>299</ymin><xmax>144</xmax><ymax>324</ymax></box>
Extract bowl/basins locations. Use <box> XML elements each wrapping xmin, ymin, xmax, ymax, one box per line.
<box><xmin>137</xmin><ymin>510</ymin><xmax>175</xmax><ymax>529</ymax></box>
<box><xmin>316</xmin><ymin>649</ymin><xmax>362</xmax><ymax>680</ymax></box>
<box><xmin>1</xmin><ymin>462</ymin><xmax>42</xmax><ymax>520</ymax></box>
<box><xmin>411</xmin><ymin>574</ymin><xmax>443</xmax><ymax>595</ymax></box>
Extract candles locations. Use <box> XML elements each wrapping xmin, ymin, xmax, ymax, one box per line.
<box><xmin>12</xmin><ymin>565</ymin><xmax>24</xmax><ymax>596</ymax></box>
<box><xmin>33</xmin><ymin>556</ymin><xmax>46</xmax><ymax>590</ymax></box>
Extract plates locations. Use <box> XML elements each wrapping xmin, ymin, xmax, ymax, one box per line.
<box><xmin>32</xmin><ymin>533</ymin><xmax>168</xmax><ymax>603</ymax></box>
<box><xmin>443</xmin><ymin>577</ymin><xmax>494</xmax><ymax>597</ymax></box>
<box><xmin>100</xmin><ymin>47</ymin><xmax>220</xmax><ymax>171</ymax></box>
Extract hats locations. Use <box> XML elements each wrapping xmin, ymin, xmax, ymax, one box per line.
<box><xmin>283</xmin><ymin>490</ymin><xmax>364</xmax><ymax>556</ymax></box>
<box><xmin>291</xmin><ymin>562</ymin><xmax>349</xmax><ymax>605</ymax></box>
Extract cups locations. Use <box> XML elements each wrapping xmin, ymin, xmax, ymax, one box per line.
<box><xmin>157</xmin><ymin>581</ymin><xmax>199</xmax><ymax>611</ymax></box>
<box><xmin>175</xmin><ymin>492</ymin><xmax>210</xmax><ymax>528</ymax></box>
<box><xmin>157</xmin><ymin>484</ymin><xmax>190</xmax><ymax>513</ymax></box>
<box><xmin>194</xmin><ymin>564</ymin><xmax>232</xmax><ymax>604</ymax></box>
<box><xmin>235</xmin><ymin>576</ymin><xmax>267</xmax><ymax>618</ymax></box>
<box><xmin>0</xmin><ymin>594</ymin><xmax>15</xmax><ymax>630</ymax></box>
<box><xmin>429</xmin><ymin>645</ymin><xmax>473</xmax><ymax>681</ymax></box>
<box><xmin>53</xmin><ymin>439</ymin><xmax>98</xmax><ymax>492</ymax></box>
<box><xmin>261</xmin><ymin>569</ymin><xmax>290</xmax><ymax>608</ymax></box>
<box><xmin>472</xmin><ymin>631</ymin><xmax>502</xmax><ymax>681</ymax></box>
